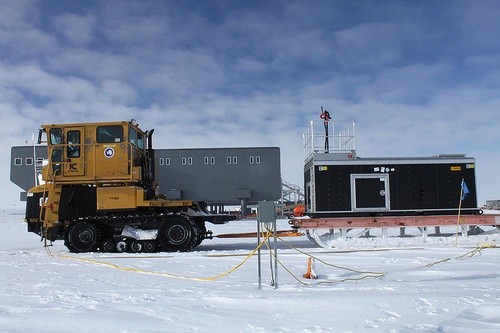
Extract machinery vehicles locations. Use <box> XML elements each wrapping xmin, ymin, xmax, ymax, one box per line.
<box><xmin>10</xmin><ymin>119</ymin><xmax>282</xmax><ymax>253</ymax></box>
